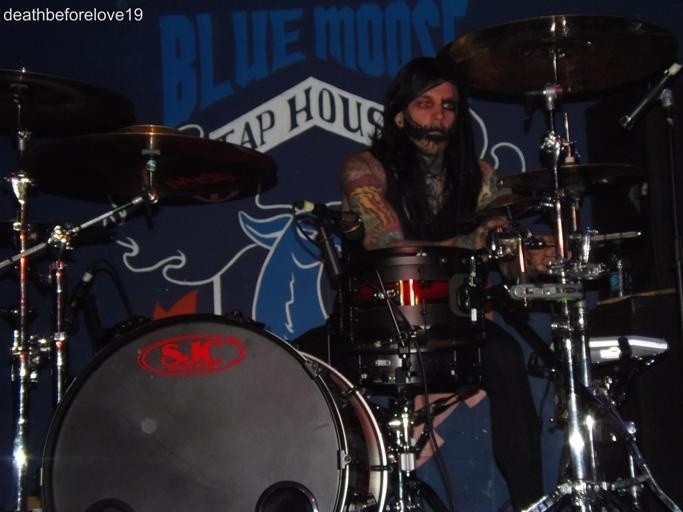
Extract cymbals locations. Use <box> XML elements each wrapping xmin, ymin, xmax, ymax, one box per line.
<box><xmin>437</xmin><ymin>14</ymin><xmax>671</xmax><ymax>102</ymax></box>
<box><xmin>0</xmin><ymin>67</ymin><xmax>132</xmax><ymax>133</ymax></box>
<box><xmin>499</xmin><ymin>162</ymin><xmax>641</xmax><ymax>189</ymax></box>
<box><xmin>30</xmin><ymin>124</ymin><xmax>277</xmax><ymax>205</ymax></box>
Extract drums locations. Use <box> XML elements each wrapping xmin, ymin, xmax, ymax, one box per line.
<box><xmin>39</xmin><ymin>313</ymin><xmax>388</xmax><ymax>509</ymax></box>
<box><xmin>349</xmin><ymin>244</ymin><xmax>484</xmax><ymax>394</ymax></box>
<box><xmin>546</xmin><ymin>230</ymin><xmax>670</xmax><ymax>364</ymax></box>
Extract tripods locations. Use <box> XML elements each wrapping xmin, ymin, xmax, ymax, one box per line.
<box><xmin>385</xmin><ymin>196</ymin><xmax>682</xmax><ymax>512</ymax></box>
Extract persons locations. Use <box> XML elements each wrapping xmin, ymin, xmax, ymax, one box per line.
<box><xmin>332</xmin><ymin>50</ymin><xmax>577</xmax><ymax>512</ymax></box>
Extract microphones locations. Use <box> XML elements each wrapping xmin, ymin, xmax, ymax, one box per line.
<box><xmin>296</xmin><ymin>200</ymin><xmax>363</xmax><ymax>233</ymax></box>
<box><xmin>618</xmin><ymin>62</ymin><xmax>683</xmax><ymax>130</ymax></box>
<box><xmin>57</xmin><ymin>261</ymin><xmax>103</xmax><ymax>338</ymax></box>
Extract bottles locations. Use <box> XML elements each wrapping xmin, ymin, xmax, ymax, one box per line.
<box><xmin>606</xmin><ymin>240</ymin><xmax>633</xmax><ymax>298</ymax></box>
<box><xmin>26</xmin><ymin>472</ymin><xmax>40</xmax><ymax>511</ymax></box>
